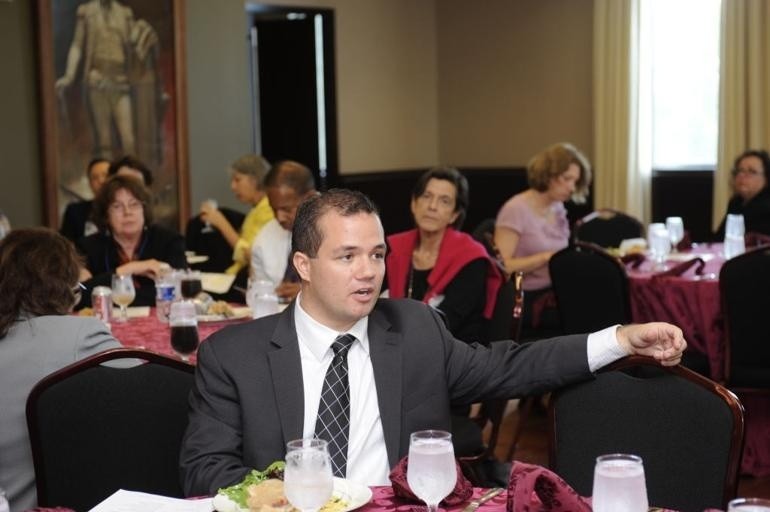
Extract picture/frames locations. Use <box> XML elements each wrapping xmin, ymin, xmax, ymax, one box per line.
<box><xmin>35</xmin><ymin>0</ymin><xmax>191</xmax><ymax>254</ymax></box>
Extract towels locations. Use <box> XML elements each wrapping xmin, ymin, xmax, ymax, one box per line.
<box><xmin>507</xmin><ymin>460</ymin><xmax>593</xmax><ymax>512</ymax></box>
<box><xmin>389</xmin><ymin>452</ymin><xmax>472</xmax><ymax>512</ymax></box>
<box><xmin>653</xmin><ymin>258</ymin><xmax>705</xmax><ymax>278</ymax></box>
<box><xmin>620</xmin><ymin>252</ymin><xmax>643</xmax><ymax>273</ymax></box>
<box><xmin>89</xmin><ymin>490</ymin><xmax>218</xmax><ymax>512</ymax></box>
<box><xmin>677</xmin><ymin>232</ymin><xmax>694</xmax><ymax>253</ymax></box>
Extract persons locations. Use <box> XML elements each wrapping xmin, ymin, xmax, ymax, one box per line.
<box><xmin>55</xmin><ymin>0</ymin><xmax>165</xmax><ymax>157</ymax></box>
<box><xmin>711</xmin><ymin>150</ymin><xmax>770</xmax><ymax>244</ymax></box>
<box><xmin>201</xmin><ymin>158</ymin><xmax>316</xmax><ymax>315</ymax></box>
<box><xmin>56</xmin><ymin>156</ymin><xmax>190</xmax><ymax>309</ymax></box>
<box><xmin>496</xmin><ymin>143</ymin><xmax>593</xmax><ymax>292</ymax></box>
<box><xmin>175</xmin><ymin>188</ymin><xmax>691</xmax><ymax>497</ymax></box>
<box><xmin>386</xmin><ymin>165</ymin><xmax>505</xmax><ymax>335</ymax></box>
<box><xmin>2</xmin><ymin>228</ymin><xmax>145</xmax><ymax>512</ymax></box>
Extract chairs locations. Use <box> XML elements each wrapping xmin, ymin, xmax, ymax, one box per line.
<box><xmin>473</xmin><ymin>226</ymin><xmax>508</xmax><ymax>280</ymax></box>
<box><xmin>450</xmin><ymin>271</ymin><xmax>526</xmax><ymax>487</ymax></box>
<box><xmin>554</xmin><ymin>246</ymin><xmax>630</xmax><ymax>335</ymax></box>
<box><xmin>570</xmin><ymin>207</ymin><xmax>644</xmax><ymax>246</ymax></box>
<box><xmin>184</xmin><ymin>207</ymin><xmax>246</xmax><ymax>273</ymax></box>
<box><xmin>26</xmin><ymin>347</ymin><xmax>195</xmax><ymax>511</ymax></box>
<box><xmin>709</xmin><ymin>242</ymin><xmax>770</xmax><ymax>393</ymax></box>
<box><xmin>547</xmin><ymin>355</ymin><xmax>748</xmax><ymax>512</ymax></box>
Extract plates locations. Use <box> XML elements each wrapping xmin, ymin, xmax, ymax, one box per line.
<box><xmin>214</xmin><ymin>474</ymin><xmax>372</xmax><ymax>511</ymax></box>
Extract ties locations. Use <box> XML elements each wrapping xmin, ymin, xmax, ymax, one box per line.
<box><xmin>311</xmin><ymin>335</ymin><xmax>356</xmax><ymax>478</ymax></box>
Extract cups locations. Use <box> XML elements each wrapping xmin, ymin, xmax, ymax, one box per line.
<box><xmin>92</xmin><ymin>286</ymin><xmax>113</xmax><ymax>329</ymax></box>
<box><xmin>181</xmin><ymin>269</ymin><xmax>203</xmax><ymax>302</ymax></box>
<box><xmin>590</xmin><ymin>453</ymin><xmax>650</xmax><ymax>511</ymax></box>
<box><xmin>245</xmin><ymin>278</ymin><xmax>280</xmax><ymax>319</ymax></box>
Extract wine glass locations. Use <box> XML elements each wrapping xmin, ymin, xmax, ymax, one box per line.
<box><xmin>109</xmin><ymin>272</ymin><xmax>137</xmax><ymax>327</ymax></box>
<box><xmin>283</xmin><ymin>438</ymin><xmax>335</xmax><ymax>512</ymax></box>
<box><xmin>201</xmin><ymin>198</ymin><xmax>219</xmax><ymax>233</ymax></box>
<box><xmin>168</xmin><ymin>300</ymin><xmax>199</xmax><ymax>365</ymax></box>
<box><xmin>647</xmin><ymin>212</ymin><xmax>746</xmax><ymax>273</ymax></box>
<box><xmin>405</xmin><ymin>428</ymin><xmax>458</xmax><ymax>512</ymax></box>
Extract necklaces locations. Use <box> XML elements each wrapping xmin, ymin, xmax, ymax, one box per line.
<box><xmin>536</xmin><ymin>200</ymin><xmax>552</xmax><ymax>221</ymax></box>
<box><xmin>407</xmin><ymin>261</ymin><xmax>431</xmax><ymax>302</ymax></box>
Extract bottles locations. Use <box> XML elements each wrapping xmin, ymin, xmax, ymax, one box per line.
<box><xmin>154</xmin><ymin>264</ymin><xmax>175</xmax><ymax>324</ymax></box>
